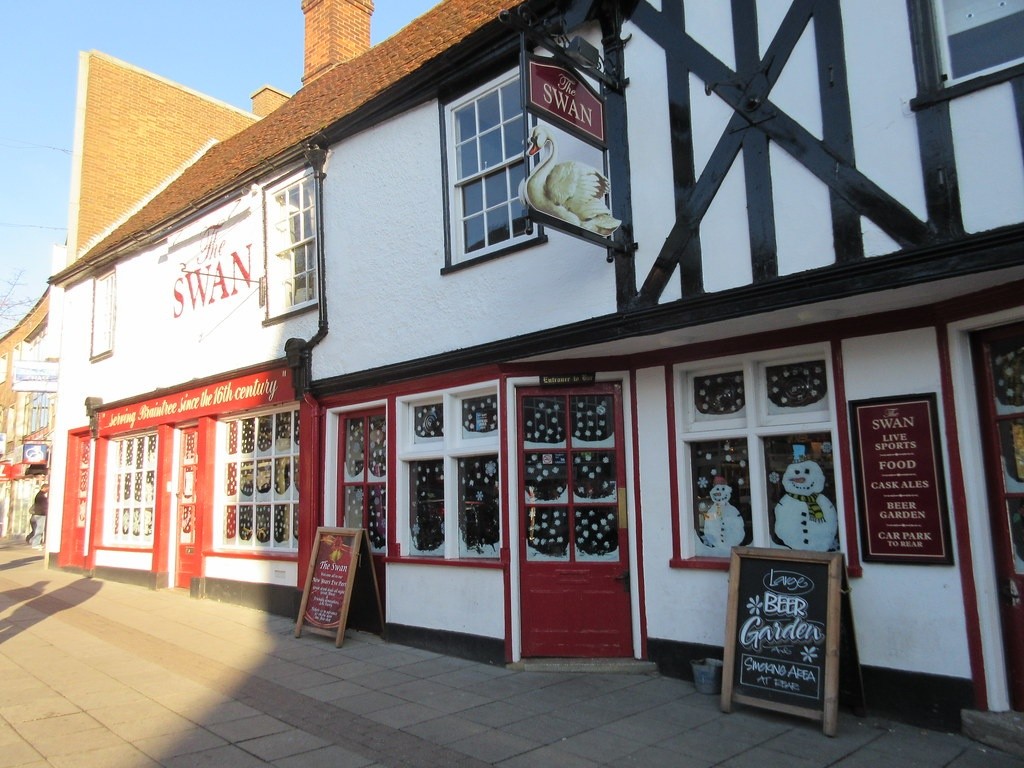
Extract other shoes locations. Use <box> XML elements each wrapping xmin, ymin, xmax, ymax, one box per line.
<box><xmin>26</xmin><ymin>537</ymin><xmax>30</xmax><ymax>544</ymax></box>
<box><xmin>33</xmin><ymin>544</ymin><xmax>45</xmax><ymax>550</ymax></box>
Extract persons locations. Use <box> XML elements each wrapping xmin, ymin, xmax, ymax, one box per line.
<box><xmin>25</xmin><ymin>484</ymin><xmax>48</xmax><ymax>549</ymax></box>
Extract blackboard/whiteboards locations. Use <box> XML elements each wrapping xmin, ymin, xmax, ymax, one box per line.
<box><xmin>721</xmin><ymin>545</ymin><xmax>862</xmax><ymax>721</ymax></box>
